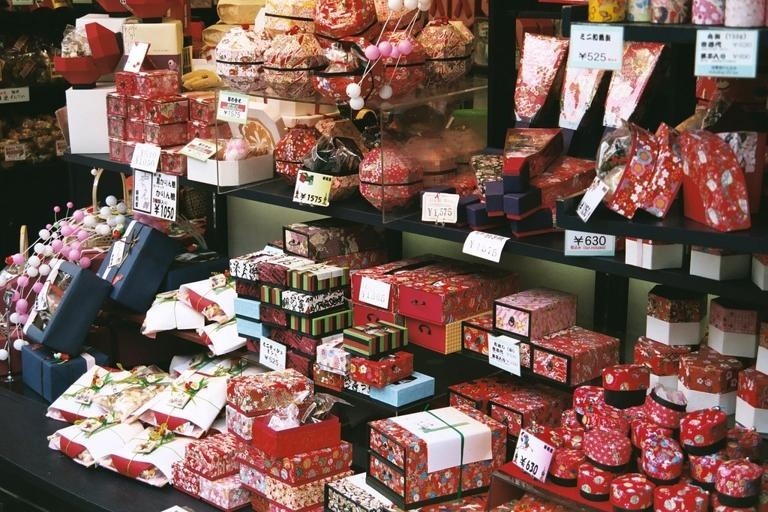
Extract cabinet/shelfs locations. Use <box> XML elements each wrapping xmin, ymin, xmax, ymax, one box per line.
<box><xmin>0</xmin><ymin>0</ymin><xmax>767</xmax><ymax>512</ymax></box>
